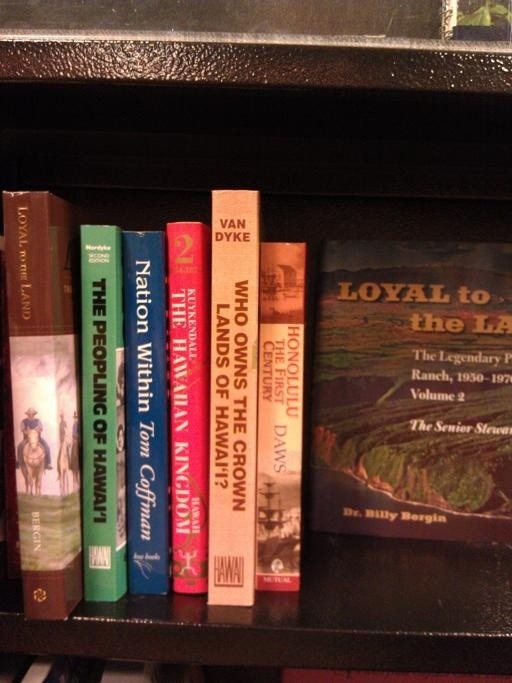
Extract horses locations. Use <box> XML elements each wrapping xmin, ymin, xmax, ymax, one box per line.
<box><xmin>23</xmin><ymin>424</ymin><xmax>47</xmax><ymax>495</ymax></box>
<box><xmin>58</xmin><ymin>424</ymin><xmax>80</xmax><ymax>498</ymax></box>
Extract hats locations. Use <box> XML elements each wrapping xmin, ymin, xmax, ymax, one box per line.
<box><xmin>25</xmin><ymin>408</ymin><xmax>37</xmax><ymax>415</ymax></box>
<box><xmin>73</xmin><ymin>412</ymin><xmax>77</xmax><ymax>418</ymax></box>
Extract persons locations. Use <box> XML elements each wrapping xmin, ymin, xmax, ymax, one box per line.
<box><xmin>0</xmin><ymin>184</ymin><xmax>511</xmax><ymax>683</ymax></box>
